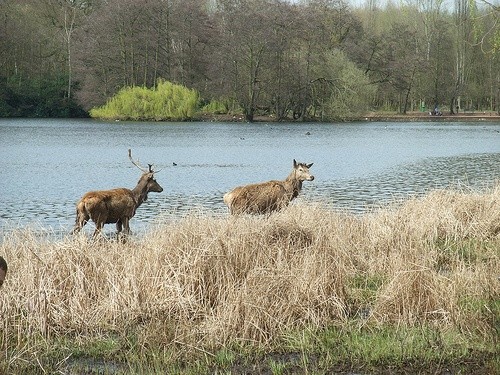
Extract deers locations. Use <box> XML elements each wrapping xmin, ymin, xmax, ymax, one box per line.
<box><xmin>66</xmin><ymin>149</ymin><xmax>164</xmax><ymax>242</ymax></box>
<box><xmin>223</xmin><ymin>158</ymin><xmax>314</xmax><ymax>216</ymax></box>
